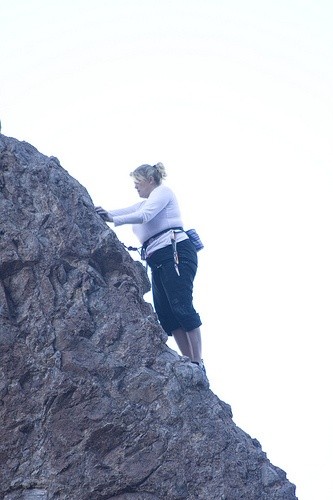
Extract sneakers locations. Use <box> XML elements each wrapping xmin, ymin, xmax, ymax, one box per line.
<box><xmin>191</xmin><ymin>361</ymin><xmax>206</xmax><ymax>375</ymax></box>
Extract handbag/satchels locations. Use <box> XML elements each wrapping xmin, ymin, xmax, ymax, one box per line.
<box><xmin>185</xmin><ymin>229</ymin><xmax>204</xmax><ymax>252</ymax></box>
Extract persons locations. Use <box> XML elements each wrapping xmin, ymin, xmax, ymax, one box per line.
<box><xmin>95</xmin><ymin>162</ymin><xmax>206</xmax><ymax>374</ymax></box>
